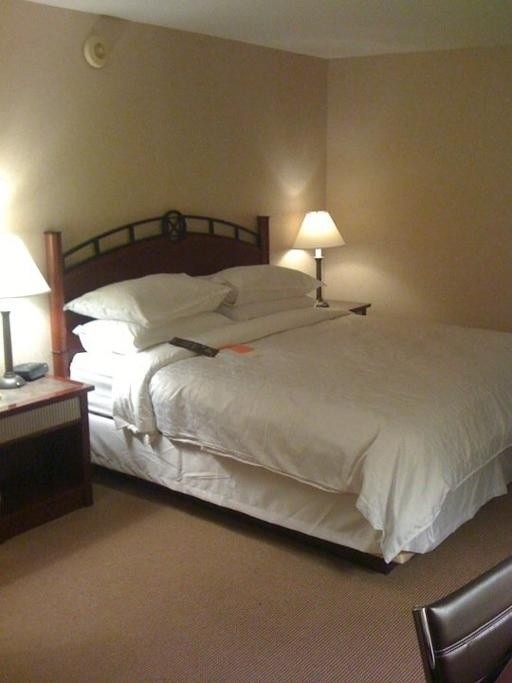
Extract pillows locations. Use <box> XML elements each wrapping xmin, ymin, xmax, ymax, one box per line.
<box><xmin>62</xmin><ymin>262</ymin><xmax>327</xmax><ymax>357</ymax></box>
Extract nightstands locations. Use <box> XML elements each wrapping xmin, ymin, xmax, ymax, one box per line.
<box><xmin>322</xmin><ymin>299</ymin><xmax>371</xmax><ymax>316</ymax></box>
<box><xmin>0</xmin><ymin>374</ymin><xmax>96</xmax><ymax>544</ymax></box>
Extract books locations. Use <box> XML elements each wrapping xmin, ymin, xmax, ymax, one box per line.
<box><xmin>9</xmin><ymin>360</ymin><xmax>51</xmax><ymax>381</ymax></box>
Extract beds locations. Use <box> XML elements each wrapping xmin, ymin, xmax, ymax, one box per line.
<box><xmin>43</xmin><ymin>208</ymin><xmax>511</xmax><ymax>575</ymax></box>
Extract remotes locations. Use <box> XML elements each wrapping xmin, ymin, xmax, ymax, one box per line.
<box><xmin>169</xmin><ymin>337</ymin><xmax>218</xmax><ymax>357</ymax></box>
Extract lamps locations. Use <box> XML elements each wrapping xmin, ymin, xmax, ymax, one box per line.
<box><xmin>0</xmin><ymin>230</ymin><xmax>53</xmax><ymax>389</ymax></box>
<box><xmin>291</xmin><ymin>210</ymin><xmax>346</xmax><ymax>307</ymax></box>
